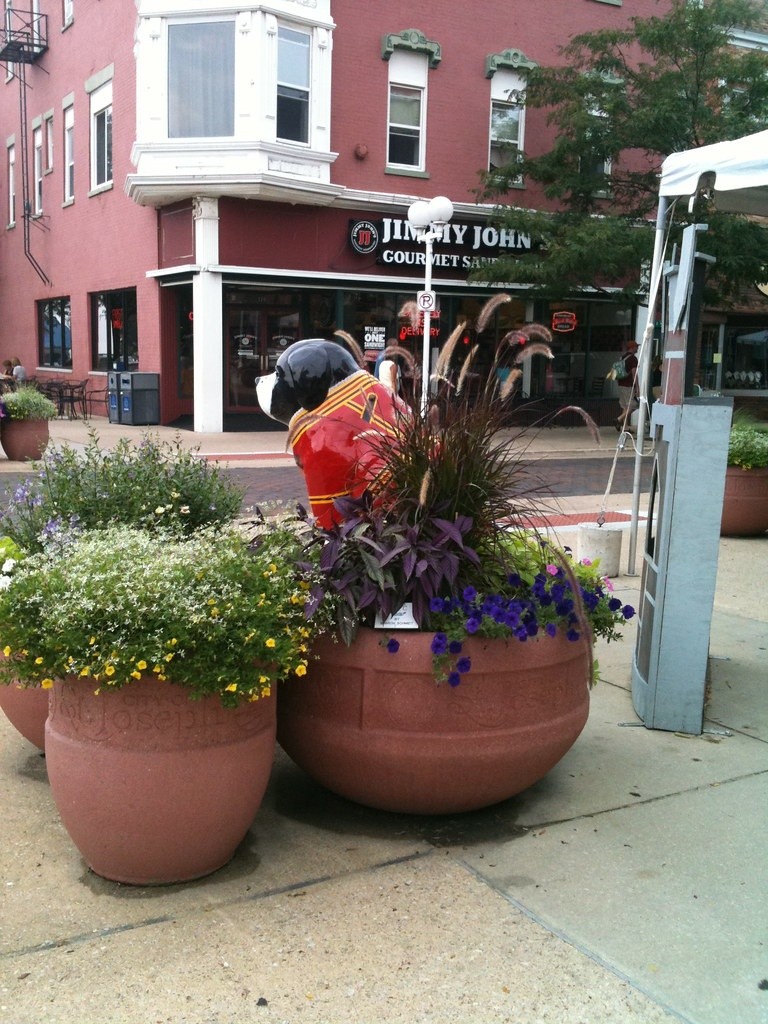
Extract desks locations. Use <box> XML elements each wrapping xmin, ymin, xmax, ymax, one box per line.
<box><xmin>47</xmin><ymin>383</ymin><xmax>79</xmax><ymax>417</ymax></box>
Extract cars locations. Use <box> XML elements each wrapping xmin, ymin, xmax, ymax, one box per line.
<box><xmin>120</xmin><ymin>356</ymin><xmax>134</xmax><ymax>362</ymax></box>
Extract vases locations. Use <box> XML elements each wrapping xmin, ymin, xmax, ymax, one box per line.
<box><xmin>292</xmin><ymin>616</ymin><xmax>598</xmax><ymax>818</ymax></box>
<box><xmin>1</xmin><ymin>648</ymin><xmax>64</xmax><ymax>744</ymax></box>
<box><xmin>721</xmin><ymin>466</ymin><xmax>768</xmax><ymax>537</ymax></box>
<box><xmin>44</xmin><ymin>648</ymin><xmax>277</xmax><ymax>886</ymax></box>
<box><xmin>0</xmin><ymin>415</ymin><xmax>51</xmax><ymax>461</ymax></box>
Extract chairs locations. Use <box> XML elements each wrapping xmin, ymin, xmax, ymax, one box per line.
<box><xmin>11</xmin><ymin>373</ymin><xmax>112</xmax><ymax>419</ymax></box>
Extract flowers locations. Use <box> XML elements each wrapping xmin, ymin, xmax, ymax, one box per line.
<box><xmin>0</xmin><ymin>386</ymin><xmax>58</xmax><ymax>418</ymax></box>
<box><xmin>0</xmin><ymin>420</ymin><xmax>325</xmax><ymax>691</ymax></box>
<box><xmin>726</xmin><ymin>420</ymin><xmax>768</xmax><ymax>470</ymax></box>
<box><xmin>302</xmin><ymin>292</ymin><xmax>636</xmax><ymax>696</ymax></box>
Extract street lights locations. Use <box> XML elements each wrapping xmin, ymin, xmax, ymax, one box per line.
<box><xmin>409</xmin><ymin>196</ymin><xmax>455</xmax><ymax>419</ymax></box>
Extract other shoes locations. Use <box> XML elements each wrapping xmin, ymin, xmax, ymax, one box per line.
<box><xmin>624</xmin><ymin>428</ymin><xmax>634</xmax><ymax>434</ymax></box>
<box><xmin>613</xmin><ymin>418</ymin><xmax>621</xmax><ymax>431</ymax></box>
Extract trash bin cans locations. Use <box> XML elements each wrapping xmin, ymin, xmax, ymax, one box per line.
<box><xmin>120</xmin><ymin>371</ymin><xmax>160</xmax><ymax>424</ymax></box>
<box><xmin>107</xmin><ymin>372</ymin><xmax>120</xmax><ymax>421</ymax></box>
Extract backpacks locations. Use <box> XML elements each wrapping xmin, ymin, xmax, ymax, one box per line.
<box><xmin>612</xmin><ymin>354</ymin><xmax>631</xmax><ymax>379</ymax></box>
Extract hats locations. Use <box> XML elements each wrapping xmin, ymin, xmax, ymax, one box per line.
<box><xmin>627</xmin><ymin>341</ymin><xmax>641</xmax><ymax>347</ymax></box>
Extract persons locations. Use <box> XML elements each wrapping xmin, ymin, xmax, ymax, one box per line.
<box><xmin>378</xmin><ymin>355</ymin><xmax>400</xmax><ymax>395</ymax></box>
<box><xmin>1</xmin><ymin>356</ymin><xmax>27</xmax><ymax>391</ymax></box>
<box><xmin>613</xmin><ymin>340</ymin><xmax>641</xmax><ymax>434</ymax></box>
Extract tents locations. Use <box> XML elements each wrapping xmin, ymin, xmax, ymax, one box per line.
<box><xmin>624</xmin><ymin>128</ymin><xmax>768</xmax><ymax>578</ymax></box>
